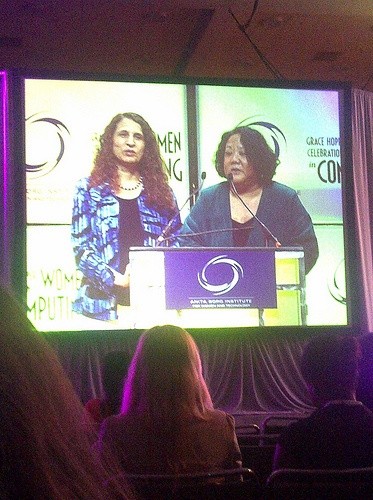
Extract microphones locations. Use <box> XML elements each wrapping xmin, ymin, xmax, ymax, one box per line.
<box><xmin>157</xmin><ymin>226</ymin><xmax>256</xmax><ymax>245</ymax></box>
<box><xmin>228</xmin><ymin>172</ymin><xmax>283</xmax><ymax>247</ymax></box>
<box><xmin>155</xmin><ymin>172</ymin><xmax>207</xmax><ymax>246</ymax></box>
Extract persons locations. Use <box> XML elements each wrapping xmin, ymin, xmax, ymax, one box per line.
<box><xmin>270</xmin><ymin>334</ymin><xmax>373</xmax><ymax>482</ymax></box>
<box><xmin>356</xmin><ymin>333</ymin><xmax>373</xmax><ymax>411</ymax></box>
<box><xmin>0</xmin><ymin>286</ymin><xmax>137</xmax><ymax>500</ymax></box>
<box><xmin>170</xmin><ymin>126</ymin><xmax>320</xmax><ymax>276</ymax></box>
<box><xmin>69</xmin><ymin>113</ymin><xmax>184</xmax><ymax>322</ymax></box>
<box><xmin>95</xmin><ymin>325</ymin><xmax>244</xmax><ymax>490</ymax></box>
<box><xmin>84</xmin><ymin>350</ymin><xmax>133</xmax><ymax>423</ymax></box>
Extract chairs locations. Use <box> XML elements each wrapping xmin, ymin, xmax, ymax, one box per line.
<box><xmin>121</xmin><ymin>416</ymin><xmax>373</xmax><ymax>500</ymax></box>
